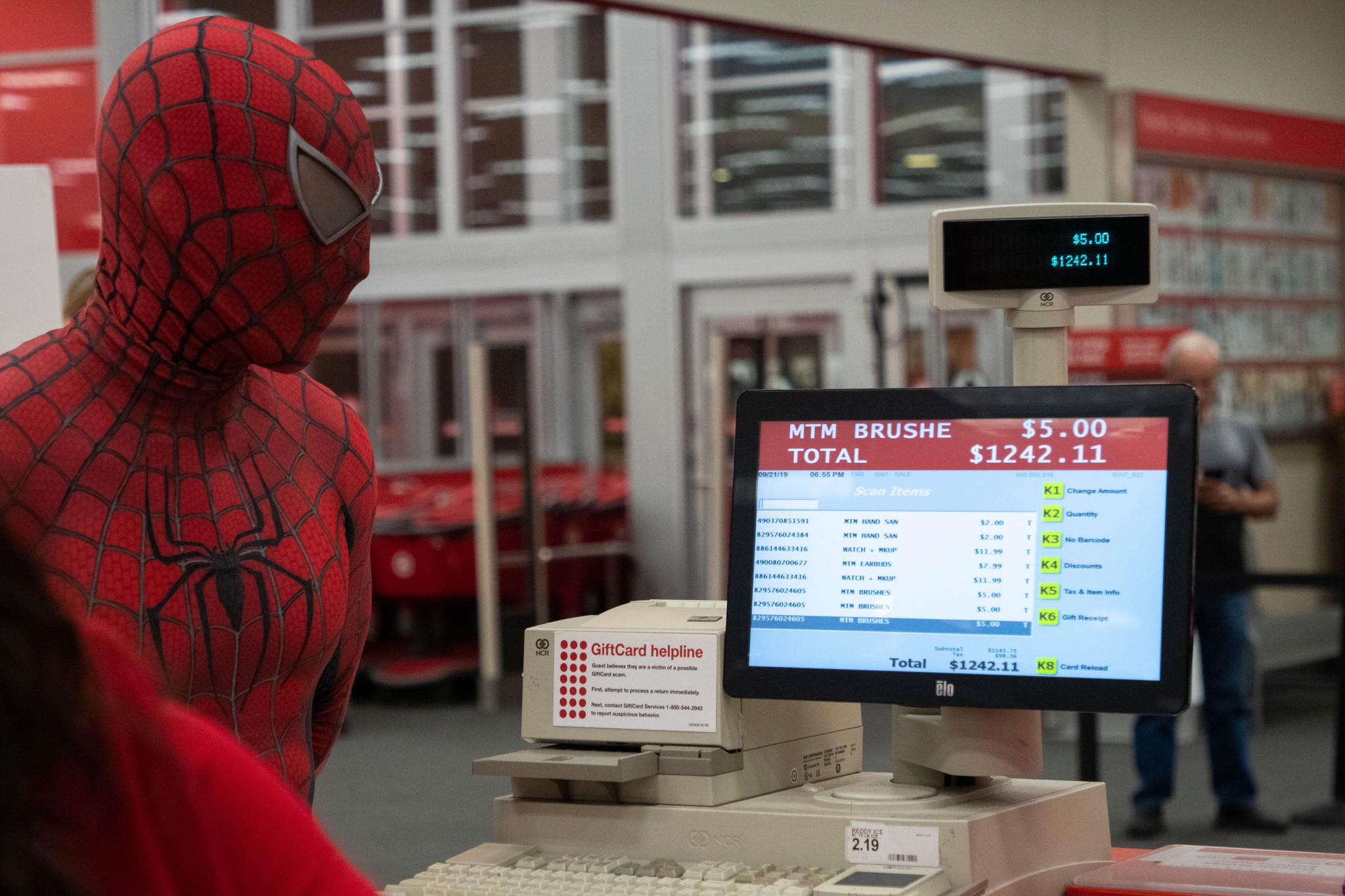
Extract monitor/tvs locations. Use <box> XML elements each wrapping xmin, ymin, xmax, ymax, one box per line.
<box><xmin>722</xmin><ymin>377</ymin><xmax>1205</xmax><ymax>787</ymax></box>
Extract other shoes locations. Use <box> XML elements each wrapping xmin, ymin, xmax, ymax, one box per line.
<box><xmin>1211</xmin><ymin>805</ymin><xmax>1289</xmax><ymax>834</ymax></box>
<box><xmin>1126</xmin><ymin>815</ymin><xmax>1163</xmax><ymax>840</ymax></box>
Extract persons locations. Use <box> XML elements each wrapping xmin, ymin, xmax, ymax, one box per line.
<box><xmin>0</xmin><ymin>526</ymin><xmax>377</xmax><ymax>896</ymax></box>
<box><xmin>0</xmin><ymin>16</ymin><xmax>382</xmax><ymax>820</ymax></box>
<box><xmin>1290</xmin><ymin>413</ymin><xmax>1345</xmax><ymax>829</ymax></box>
<box><xmin>1122</xmin><ymin>331</ymin><xmax>1287</xmax><ymax>838</ymax></box>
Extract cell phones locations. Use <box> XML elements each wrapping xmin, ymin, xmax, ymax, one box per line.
<box><xmin>1208</xmin><ymin>468</ymin><xmax>1226</xmax><ymax>479</ymax></box>
